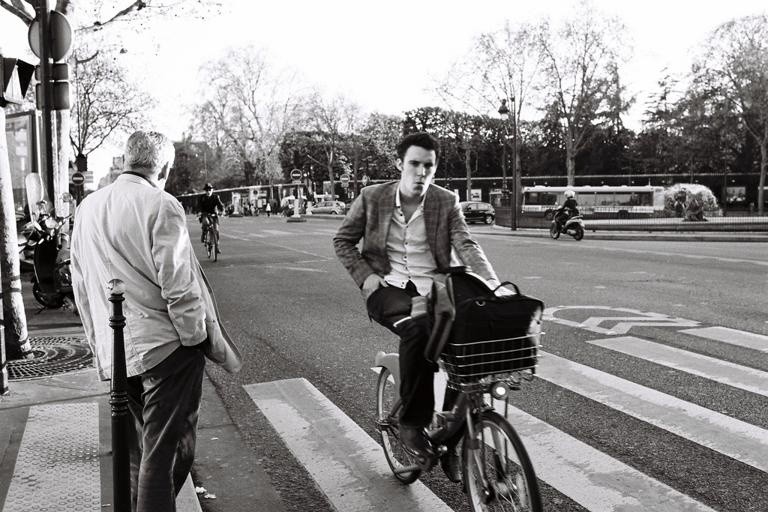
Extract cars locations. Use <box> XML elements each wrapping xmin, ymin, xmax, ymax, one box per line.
<box><xmin>285</xmin><ymin>199</ymin><xmax>345</xmax><ymax>217</ymax></box>
<box><xmin>460</xmin><ymin>201</ymin><xmax>496</xmax><ymax>224</ymax></box>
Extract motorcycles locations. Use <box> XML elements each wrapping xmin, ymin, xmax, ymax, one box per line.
<box><xmin>17</xmin><ymin>199</ymin><xmax>78</xmax><ymax>317</ymax></box>
<box><xmin>550</xmin><ymin>209</ymin><xmax>585</xmax><ymax>240</ymax></box>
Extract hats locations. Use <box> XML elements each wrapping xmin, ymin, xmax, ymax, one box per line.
<box><xmin>202</xmin><ymin>182</ymin><xmax>216</xmax><ymax>190</ymax></box>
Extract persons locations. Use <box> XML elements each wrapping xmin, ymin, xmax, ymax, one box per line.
<box><xmin>68</xmin><ymin>132</ymin><xmax>247</xmax><ymax>511</ymax></box>
<box><xmin>553</xmin><ymin>190</ymin><xmax>580</xmax><ymax>231</ymax></box>
<box><xmin>673</xmin><ymin>186</ymin><xmax>688</xmax><ymax>217</ymax></box>
<box><xmin>195</xmin><ymin>182</ymin><xmax>228</xmax><ymax>254</ymax></box>
<box><xmin>332</xmin><ymin>134</ymin><xmax>504</xmax><ymax>485</ymax></box>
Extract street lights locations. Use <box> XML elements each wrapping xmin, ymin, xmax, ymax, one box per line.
<box><xmin>498</xmin><ymin>96</ymin><xmax>516</xmax><ymax>230</ymax></box>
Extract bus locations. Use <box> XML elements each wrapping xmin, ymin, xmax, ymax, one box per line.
<box><xmin>521</xmin><ymin>185</ymin><xmax>665</xmax><ymax>219</ymax></box>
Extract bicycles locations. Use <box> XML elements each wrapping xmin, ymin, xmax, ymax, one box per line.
<box><xmin>374</xmin><ymin>297</ymin><xmax>546</xmax><ymax>512</ymax></box>
<box><xmin>198</xmin><ymin>211</ymin><xmax>226</xmax><ymax>261</ymax></box>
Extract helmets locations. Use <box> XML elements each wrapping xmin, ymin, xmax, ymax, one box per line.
<box><xmin>564</xmin><ymin>190</ymin><xmax>575</xmax><ymax>199</ymax></box>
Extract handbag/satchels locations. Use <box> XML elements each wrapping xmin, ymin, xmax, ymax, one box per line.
<box><xmin>444</xmin><ymin>278</ymin><xmax>545</xmax><ymax>373</ymax></box>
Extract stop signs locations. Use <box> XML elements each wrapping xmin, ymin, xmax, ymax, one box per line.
<box><xmin>362</xmin><ymin>175</ymin><xmax>367</xmax><ymax>186</ymax></box>
<box><xmin>340</xmin><ymin>174</ymin><xmax>350</xmax><ymax>184</ymax></box>
<box><xmin>71</xmin><ymin>172</ymin><xmax>86</xmax><ymax>186</ymax></box>
<box><xmin>291</xmin><ymin>169</ymin><xmax>302</xmax><ymax>181</ymax></box>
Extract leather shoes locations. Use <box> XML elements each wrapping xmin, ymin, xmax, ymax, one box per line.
<box><xmin>399</xmin><ymin>419</ymin><xmax>429</xmax><ymax>465</ymax></box>
<box><xmin>440</xmin><ymin>447</ymin><xmax>463</xmax><ymax>483</ymax></box>
